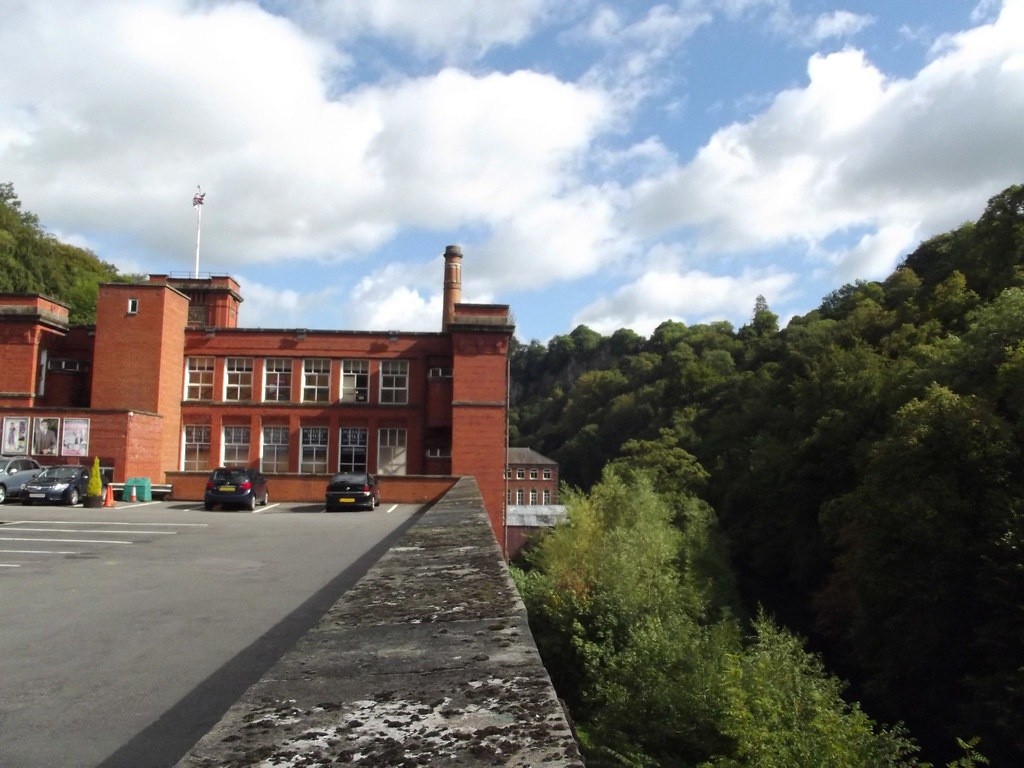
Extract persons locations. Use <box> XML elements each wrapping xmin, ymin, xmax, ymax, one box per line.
<box><xmin>8</xmin><ymin>422</ymin><xmax>18</xmax><ymax>450</ymax></box>
<box><xmin>36</xmin><ymin>419</ymin><xmax>56</xmax><ymax>455</ymax></box>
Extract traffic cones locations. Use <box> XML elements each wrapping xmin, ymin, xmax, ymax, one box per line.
<box><xmin>103</xmin><ymin>485</ymin><xmax>117</xmax><ymax>506</ymax></box>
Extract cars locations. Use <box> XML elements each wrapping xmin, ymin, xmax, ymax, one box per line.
<box><xmin>325</xmin><ymin>472</ymin><xmax>380</xmax><ymax>511</ymax></box>
<box><xmin>203</xmin><ymin>467</ymin><xmax>269</xmax><ymax>510</ymax></box>
<box><xmin>0</xmin><ymin>454</ymin><xmax>43</xmax><ymax>505</ymax></box>
<box><xmin>20</xmin><ymin>464</ymin><xmax>93</xmax><ymax>505</ymax></box>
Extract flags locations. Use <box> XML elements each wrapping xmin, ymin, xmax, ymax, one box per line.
<box><xmin>193</xmin><ymin>193</ymin><xmax>206</xmax><ymax>208</ymax></box>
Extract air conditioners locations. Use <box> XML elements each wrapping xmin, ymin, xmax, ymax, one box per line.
<box><xmin>430</xmin><ymin>367</ymin><xmax>442</xmax><ymax>377</ymax></box>
<box><xmin>427</xmin><ymin>448</ymin><xmax>440</xmax><ymax>458</ymax></box>
